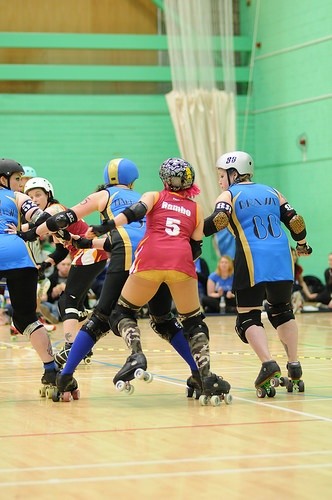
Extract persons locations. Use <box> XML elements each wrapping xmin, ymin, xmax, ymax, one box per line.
<box><xmin>86</xmin><ymin>157</ymin><xmax>232</xmax><ymax>408</ymax></box>
<box><xmin>202</xmin><ymin>151</ymin><xmax>312</xmax><ymax>398</ymax></box>
<box><xmin>0</xmin><ymin>158</ymin><xmax>332</xmax><ymax>400</ymax></box>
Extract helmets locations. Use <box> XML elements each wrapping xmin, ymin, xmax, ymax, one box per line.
<box><xmin>104</xmin><ymin>158</ymin><xmax>139</xmax><ymax>185</ymax></box>
<box><xmin>215</xmin><ymin>152</ymin><xmax>254</xmax><ymax>179</ymax></box>
<box><xmin>0</xmin><ymin>158</ymin><xmax>25</xmax><ymax>178</ymax></box>
<box><xmin>21</xmin><ymin>166</ymin><xmax>37</xmax><ymax>179</ymax></box>
<box><xmin>24</xmin><ymin>177</ymin><xmax>54</xmax><ymax>198</ymax></box>
<box><xmin>159</xmin><ymin>158</ymin><xmax>194</xmax><ymax>192</ymax></box>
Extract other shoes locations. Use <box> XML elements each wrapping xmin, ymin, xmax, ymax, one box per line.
<box><xmin>39</xmin><ymin>304</ymin><xmax>58</xmax><ymax>324</ymax></box>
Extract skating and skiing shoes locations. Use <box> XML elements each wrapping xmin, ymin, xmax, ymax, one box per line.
<box><xmin>198</xmin><ymin>374</ymin><xmax>232</xmax><ymax>406</ymax></box>
<box><xmin>287</xmin><ymin>361</ymin><xmax>304</xmax><ymax>392</ymax></box>
<box><xmin>39</xmin><ymin>368</ymin><xmax>61</xmax><ymax>399</ymax></box>
<box><xmin>255</xmin><ymin>360</ymin><xmax>289</xmax><ymax>398</ymax></box>
<box><xmin>113</xmin><ymin>353</ymin><xmax>153</xmax><ymax>394</ymax></box>
<box><xmin>187</xmin><ymin>374</ymin><xmax>204</xmax><ymax>399</ymax></box>
<box><xmin>53</xmin><ymin>341</ymin><xmax>93</xmax><ymax>370</ymax></box>
<box><xmin>10</xmin><ymin>313</ymin><xmax>57</xmax><ymax>342</ymax></box>
<box><xmin>51</xmin><ymin>372</ymin><xmax>79</xmax><ymax>402</ymax></box>
